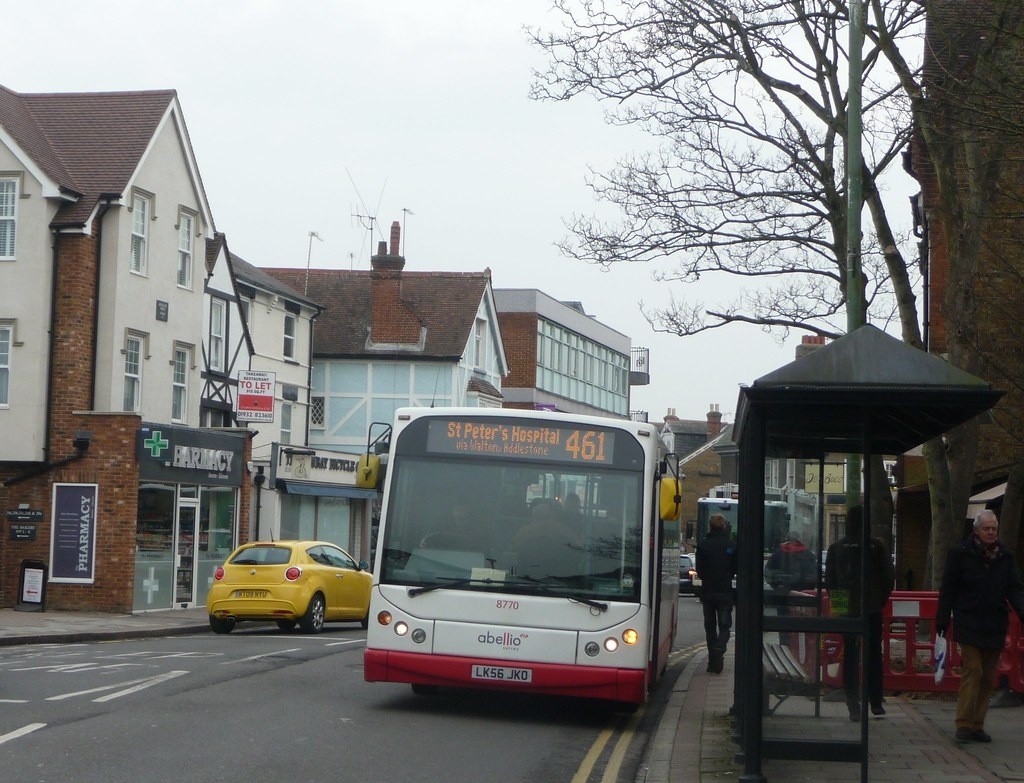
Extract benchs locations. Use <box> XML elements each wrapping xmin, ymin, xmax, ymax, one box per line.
<box><xmin>762</xmin><ymin>641</ymin><xmax>822</xmax><ymax>718</ymax></box>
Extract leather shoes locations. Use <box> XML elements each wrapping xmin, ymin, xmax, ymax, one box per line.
<box><xmin>969</xmin><ymin>729</ymin><xmax>992</xmax><ymax>743</ymax></box>
<box><xmin>955</xmin><ymin>727</ymin><xmax>970</xmax><ymax>743</ymax></box>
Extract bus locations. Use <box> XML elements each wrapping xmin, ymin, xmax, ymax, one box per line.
<box><xmin>356</xmin><ymin>405</ymin><xmax>682</xmax><ymax>712</ymax></box>
<box><xmin>691</xmin><ymin>499</ymin><xmax>789</xmax><ymax>602</ymax></box>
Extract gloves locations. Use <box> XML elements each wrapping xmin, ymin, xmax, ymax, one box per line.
<box><xmin>935</xmin><ymin>616</ymin><xmax>951</xmax><ymax>637</ymax></box>
<box><xmin>725</xmin><ymin>520</ymin><xmax>732</xmax><ymax>535</ymax></box>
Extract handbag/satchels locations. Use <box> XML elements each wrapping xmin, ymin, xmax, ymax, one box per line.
<box><xmin>934</xmin><ymin>630</ymin><xmax>947</xmax><ymax>686</ymax></box>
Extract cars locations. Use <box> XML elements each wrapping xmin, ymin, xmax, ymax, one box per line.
<box><xmin>680</xmin><ymin>553</ymin><xmax>695</xmax><ymax>592</ymax></box>
<box><xmin>812</xmin><ymin>551</ymin><xmax>828</xmax><ymax>586</ymax></box>
<box><xmin>207</xmin><ymin>539</ymin><xmax>374</xmax><ymax>635</ymax></box>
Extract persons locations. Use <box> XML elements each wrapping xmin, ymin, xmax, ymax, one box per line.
<box><xmin>825</xmin><ymin>504</ymin><xmax>895</xmax><ymax>722</ymax></box>
<box><xmin>934</xmin><ymin>510</ymin><xmax>1024</xmax><ymax>742</ymax></box>
<box><xmin>764</xmin><ymin>530</ymin><xmax>822</xmax><ymax>647</ymax></box>
<box><xmin>696</xmin><ymin>514</ymin><xmax>736</xmax><ymax>673</ymax></box>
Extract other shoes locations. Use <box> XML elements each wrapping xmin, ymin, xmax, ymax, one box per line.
<box><xmin>848</xmin><ymin>703</ymin><xmax>861</xmax><ymax>721</ymax></box>
<box><xmin>696</xmin><ymin>598</ymin><xmax>701</xmax><ymax>602</ymax></box>
<box><xmin>712</xmin><ymin>645</ymin><xmax>724</xmax><ymax>673</ymax></box>
<box><xmin>706</xmin><ymin>662</ymin><xmax>713</xmax><ymax>673</ymax></box>
<box><xmin>870</xmin><ymin>697</ymin><xmax>886</xmax><ymax>715</ymax></box>
